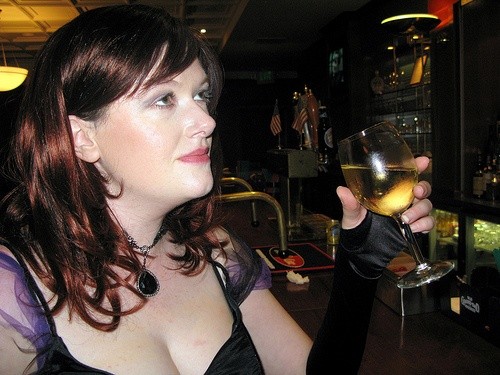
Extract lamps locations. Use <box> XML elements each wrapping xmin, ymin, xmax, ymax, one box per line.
<box><xmin>0</xmin><ymin>35</ymin><xmax>28</xmax><ymax>92</ymax></box>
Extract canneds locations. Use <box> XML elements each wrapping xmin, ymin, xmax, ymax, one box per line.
<box><xmin>326</xmin><ymin>219</ymin><xmax>341</xmax><ymax>245</ymax></box>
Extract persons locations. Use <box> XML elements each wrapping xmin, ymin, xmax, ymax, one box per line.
<box><xmin>0</xmin><ymin>5</ymin><xmax>434</xmax><ymax>375</ymax></box>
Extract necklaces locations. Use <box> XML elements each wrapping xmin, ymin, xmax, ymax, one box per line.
<box><xmin>124</xmin><ymin>223</ymin><xmax>164</xmax><ymax>296</ymax></box>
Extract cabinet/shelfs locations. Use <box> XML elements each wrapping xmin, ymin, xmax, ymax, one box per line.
<box><xmin>363</xmin><ymin>53</ymin><xmax>433</xmax><ymax>158</ymax></box>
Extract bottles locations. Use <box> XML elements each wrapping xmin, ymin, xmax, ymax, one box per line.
<box><xmin>484</xmin><ymin>155</ymin><xmax>492</xmax><ymax>187</ymax></box>
<box><xmin>318</xmin><ymin>106</ymin><xmax>334</xmax><ymax>154</ymax></box>
<box><xmin>472</xmin><ymin>150</ymin><xmax>485</xmax><ymax>199</ymax></box>
<box><xmin>491</xmin><ymin>154</ymin><xmax>500</xmax><ymax>187</ymax></box>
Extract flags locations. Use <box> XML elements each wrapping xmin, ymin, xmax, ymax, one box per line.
<box><xmin>270</xmin><ymin>103</ymin><xmax>282</xmax><ymax>136</ymax></box>
<box><xmin>292</xmin><ymin>100</ymin><xmax>308</xmax><ymax>133</ymax></box>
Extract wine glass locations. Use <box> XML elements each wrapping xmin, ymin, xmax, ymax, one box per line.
<box><xmin>336</xmin><ymin>120</ymin><xmax>456</xmax><ymax>288</ymax></box>
